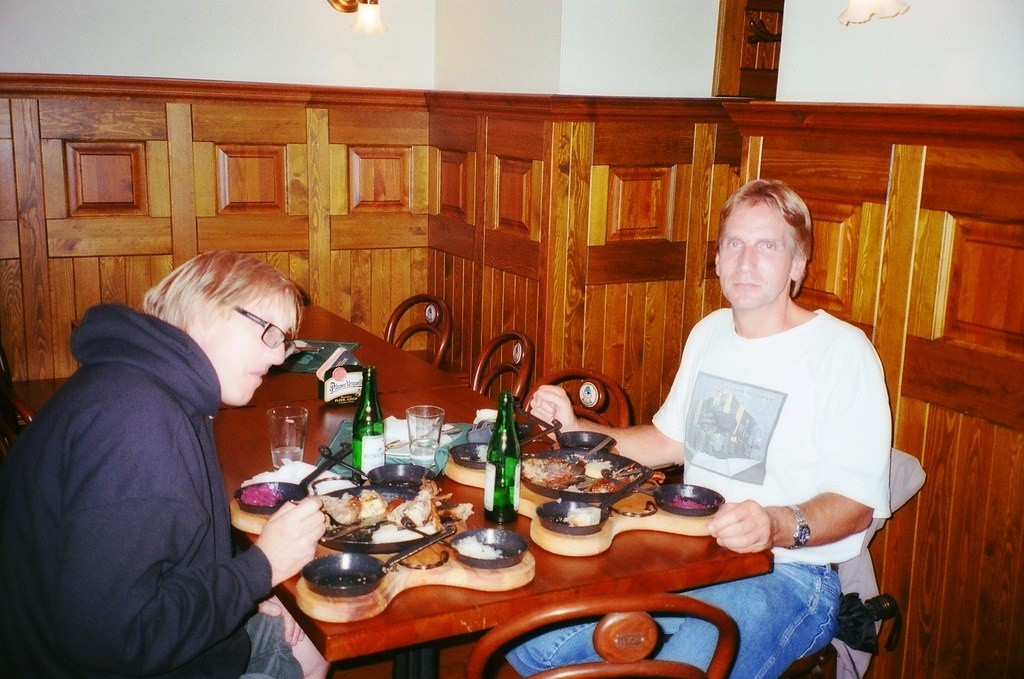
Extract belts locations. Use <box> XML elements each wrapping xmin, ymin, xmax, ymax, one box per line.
<box><xmin>822</xmin><ymin>563</ymin><xmax>839</xmax><ymax>575</ymax></box>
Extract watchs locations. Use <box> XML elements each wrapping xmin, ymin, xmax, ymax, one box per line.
<box><xmin>785</xmin><ymin>505</ymin><xmax>811</xmax><ymax>550</ymax></box>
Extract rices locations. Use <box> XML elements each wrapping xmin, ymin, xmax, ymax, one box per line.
<box><xmin>455</xmin><ymin>534</ymin><xmax>503</xmax><ymax>560</ymax></box>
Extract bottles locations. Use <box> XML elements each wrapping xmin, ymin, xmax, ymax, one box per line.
<box><xmin>484</xmin><ymin>391</ymin><xmax>522</xmax><ymax>525</ymax></box>
<box><xmin>352</xmin><ymin>364</ymin><xmax>385</xmax><ymax>486</ymax></box>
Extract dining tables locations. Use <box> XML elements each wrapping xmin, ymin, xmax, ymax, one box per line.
<box><xmin>254</xmin><ymin>302</ymin><xmax>469</xmax><ymax>404</ymax></box>
<box><xmin>206</xmin><ymin>389</ymin><xmax>775</xmax><ymax>679</ymax></box>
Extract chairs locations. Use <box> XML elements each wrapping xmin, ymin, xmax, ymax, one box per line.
<box><xmin>385</xmin><ymin>294</ymin><xmax>453</xmax><ymax>367</ymax></box>
<box><xmin>780</xmin><ymin>642</ymin><xmax>837</xmax><ymax>679</ymax></box>
<box><xmin>523</xmin><ymin>367</ymin><xmax>633</xmax><ymax>429</ymax></box>
<box><xmin>474</xmin><ymin>329</ymin><xmax>535</xmax><ymax>406</ymax></box>
<box><xmin>466</xmin><ymin>592</ymin><xmax>742</xmax><ymax>679</ymax></box>
<box><xmin>2</xmin><ymin>381</ymin><xmax>32</xmax><ymax>425</ymax></box>
<box><xmin>0</xmin><ymin>414</ymin><xmax>17</xmax><ymax>459</ymax></box>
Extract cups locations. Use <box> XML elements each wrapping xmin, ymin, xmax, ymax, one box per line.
<box><xmin>267</xmin><ymin>406</ymin><xmax>309</xmax><ymax>472</ymax></box>
<box><xmin>406</xmin><ymin>404</ymin><xmax>446</xmax><ymax>468</ymax></box>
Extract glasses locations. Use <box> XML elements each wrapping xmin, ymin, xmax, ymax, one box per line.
<box><xmin>233</xmin><ymin>305</ymin><xmax>296</xmax><ymax>360</ymax></box>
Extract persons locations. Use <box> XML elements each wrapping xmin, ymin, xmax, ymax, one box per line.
<box><xmin>504</xmin><ymin>178</ymin><xmax>892</xmax><ymax>679</ymax></box>
<box><xmin>0</xmin><ymin>250</ymin><xmax>327</xmax><ymax>679</ymax></box>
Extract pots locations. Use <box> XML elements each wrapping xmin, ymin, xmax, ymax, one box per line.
<box><xmin>601</xmin><ymin>470</ymin><xmax>724</xmax><ymax>517</ymax></box>
<box><xmin>402</xmin><ymin>515</ymin><xmax>529</xmax><ymax>568</ymax></box>
<box><xmin>513</xmin><ymin>406</ymin><xmax>618</xmax><ymax>453</ymax></box>
<box><xmin>319</xmin><ymin>438</ymin><xmax>436</xmax><ymax>490</ymax></box>
<box><xmin>301</xmin><ymin>524</ymin><xmax>457</xmax><ymax>598</ymax></box>
<box><xmin>536</xmin><ymin>465</ymin><xmax>654</xmax><ymax>535</ymax></box>
<box><xmin>233</xmin><ymin>438</ymin><xmax>351</xmax><ymax>514</ymax></box>
<box><xmin>449</xmin><ymin>417</ymin><xmax>563</xmax><ymax>470</ymax></box>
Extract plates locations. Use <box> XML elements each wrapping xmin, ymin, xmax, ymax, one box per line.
<box><xmin>518</xmin><ymin>449</ymin><xmax>647</xmax><ymax>501</ymax></box>
<box><xmin>317</xmin><ymin>486</ymin><xmax>453</xmax><ymax>555</ymax></box>
<box><xmin>379</xmin><ymin>422</ymin><xmax>453</xmax><ymax>458</ymax></box>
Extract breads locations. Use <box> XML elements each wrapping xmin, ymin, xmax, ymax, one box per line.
<box><xmin>568</xmin><ymin>506</ymin><xmax>601</xmax><ymax>527</ymax></box>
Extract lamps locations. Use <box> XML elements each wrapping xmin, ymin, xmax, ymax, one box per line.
<box><xmin>836</xmin><ymin>0</ymin><xmax>911</xmax><ymax>26</ymax></box>
<box><xmin>326</xmin><ymin>0</ymin><xmax>390</xmax><ymax>35</ymax></box>
